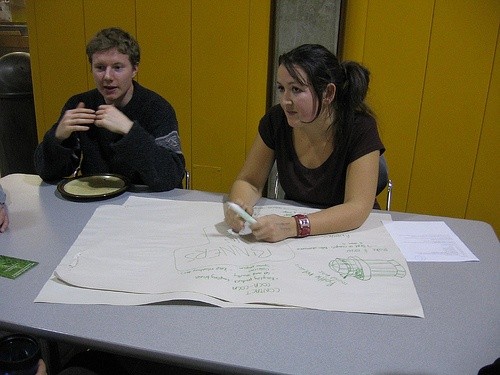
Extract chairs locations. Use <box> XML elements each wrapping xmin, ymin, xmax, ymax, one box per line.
<box><xmin>273</xmin><ymin>157</ymin><xmax>395</xmax><ymax>211</ymax></box>
<box><xmin>74</xmin><ymin>165</ymin><xmax>192</xmax><ymax>190</ymax></box>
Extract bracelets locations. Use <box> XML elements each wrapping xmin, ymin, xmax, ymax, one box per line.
<box><xmin>293</xmin><ymin>214</ymin><xmax>311</xmax><ymax>237</ymax></box>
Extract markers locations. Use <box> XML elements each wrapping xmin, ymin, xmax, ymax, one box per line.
<box><xmin>226</xmin><ymin>201</ymin><xmax>257</xmax><ymax>224</ymax></box>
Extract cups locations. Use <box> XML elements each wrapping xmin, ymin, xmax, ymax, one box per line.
<box><xmin>0</xmin><ymin>334</ymin><xmax>42</xmax><ymax>375</ymax></box>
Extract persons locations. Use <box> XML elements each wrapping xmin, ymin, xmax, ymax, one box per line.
<box><xmin>32</xmin><ymin>28</ymin><xmax>186</xmax><ymax>193</ymax></box>
<box><xmin>0</xmin><ymin>185</ymin><xmax>9</xmax><ymax>233</ymax></box>
<box><xmin>36</xmin><ymin>358</ymin><xmax>48</xmax><ymax>375</ymax></box>
<box><xmin>226</xmin><ymin>44</ymin><xmax>384</xmax><ymax>246</ymax></box>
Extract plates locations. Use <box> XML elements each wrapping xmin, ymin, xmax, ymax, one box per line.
<box><xmin>57</xmin><ymin>172</ymin><xmax>129</xmax><ymax>202</ymax></box>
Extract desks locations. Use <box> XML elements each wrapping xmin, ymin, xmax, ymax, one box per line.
<box><xmin>0</xmin><ymin>172</ymin><xmax>499</xmax><ymax>375</ymax></box>
